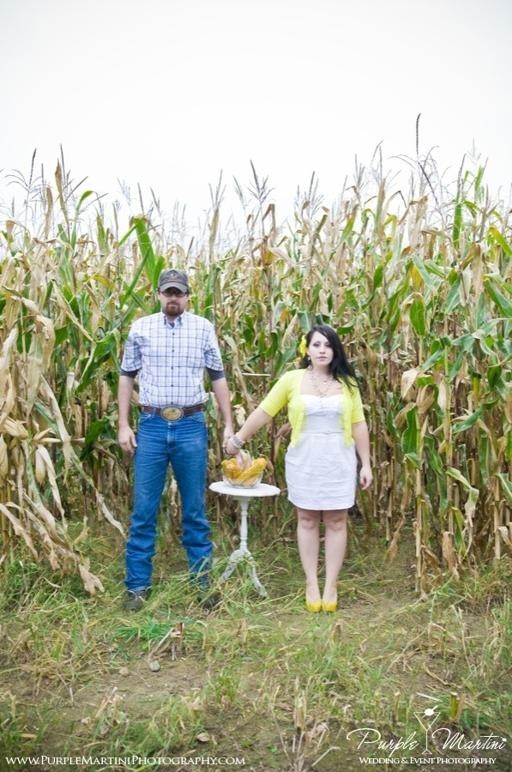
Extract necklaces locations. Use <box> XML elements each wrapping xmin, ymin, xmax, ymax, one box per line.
<box><xmin>309</xmin><ymin>369</ymin><xmax>335</xmax><ymax>398</ymax></box>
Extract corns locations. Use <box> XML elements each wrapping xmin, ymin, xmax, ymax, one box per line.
<box><xmin>0</xmin><ymin>437</ymin><xmax>8</xmax><ymax>480</ymax></box>
<box><xmin>221</xmin><ymin>457</ymin><xmax>267</xmax><ymax>489</ymax></box>
<box><xmin>35</xmin><ymin>448</ymin><xmax>45</xmax><ymax>487</ymax></box>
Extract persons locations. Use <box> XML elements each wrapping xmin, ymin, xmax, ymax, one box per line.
<box><xmin>117</xmin><ymin>269</ymin><xmax>234</xmax><ymax>613</ymax></box>
<box><xmin>224</xmin><ymin>327</ymin><xmax>374</xmax><ymax>613</ymax></box>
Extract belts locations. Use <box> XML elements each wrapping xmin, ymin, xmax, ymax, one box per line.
<box><xmin>144</xmin><ymin>405</ymin><xmax>204</xmax><ymax>424</ymax></box>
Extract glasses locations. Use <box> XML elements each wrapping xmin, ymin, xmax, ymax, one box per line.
<box><xmin>161</xmin><ymin>288</ymin><xmax>187</xmax><ymax>297</ymax></box>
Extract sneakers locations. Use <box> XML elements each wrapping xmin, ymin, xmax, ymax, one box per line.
<box><xmin>124</xmin><ymin>589</ymin><xmax>151</xmax><ymax>612</ymax></box>
<box><xmin>192</xmin><ymin>576</ymin><xmax>220</xmax><ymax>609</ymax></box>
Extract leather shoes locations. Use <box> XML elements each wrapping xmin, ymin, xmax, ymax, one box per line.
<box><xmin>306</xmin><ymin>602</ymin><xmax>321</xmax><ymax>613</ymax></box>
<box><xmin>322</xmin><ymin>602</ymin><xmax>336</xmax><ymax>613</ymax></box>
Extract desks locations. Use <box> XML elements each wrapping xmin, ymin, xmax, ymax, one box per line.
<box><xmin>208</xmin><ymin>480</ymin><xmax>281</xmax><ymax>601</ymax></box>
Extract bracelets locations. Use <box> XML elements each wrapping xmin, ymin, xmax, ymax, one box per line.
<box><xmin>232</xmin><ymin>436</ymin><xmax>247</xmax><ymax>450</ymax></box>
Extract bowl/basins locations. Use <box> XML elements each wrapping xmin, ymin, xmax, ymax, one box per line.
<box><xmin>222</xmin><ymin>468</ymin><xmax>264</xmax><ymax>489</ymax></box>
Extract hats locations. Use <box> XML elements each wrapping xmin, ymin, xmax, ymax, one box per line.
<box><xmin>158</xmin><ymin>269</ymin><xmax>188</xmax><ymax>293</ymax></box>
